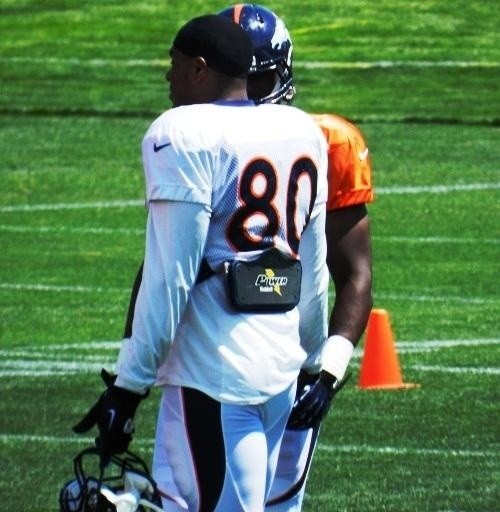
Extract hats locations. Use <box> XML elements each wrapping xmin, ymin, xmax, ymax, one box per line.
<box><xmin>172</xmin><ymin>14</ymin><xmax>255</xmax><ymax>77</ymax></box>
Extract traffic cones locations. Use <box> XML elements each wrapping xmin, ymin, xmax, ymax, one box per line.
<box><xmin>358</xmin><ymin>309</ymin><xmax>420</xmax><ymax>390</ymax></box>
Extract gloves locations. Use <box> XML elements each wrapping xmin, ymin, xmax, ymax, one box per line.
<box><xmin>73</xmin><ymin>368</ymin><xmax>152</xmax><ymax>468</ymax></box>
<box><xmin>285</xmin><ymin>334</ymin><xmax>355</xmax><ymax>431</ymax></box>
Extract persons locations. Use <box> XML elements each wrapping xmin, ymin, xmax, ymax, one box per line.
<box><xmin>112</xmin><ymin>3</ymin><xmax>375</xmax><ymax>430</ymax></box>
<box><xmin>71</xmin><ymin>14</ymin><xmax>331</xmax><ymax>512</ymax></box>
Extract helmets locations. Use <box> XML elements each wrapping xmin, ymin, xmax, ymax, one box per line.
<box><xmin>219</xmin><ymin>3</ymin><xmax>294</xmax><ymax>74</ymax></box>
<box><xmin>59</xmin><ymin>472</ymin><xmax>164</xmax><ymax>512</ymax></box>
